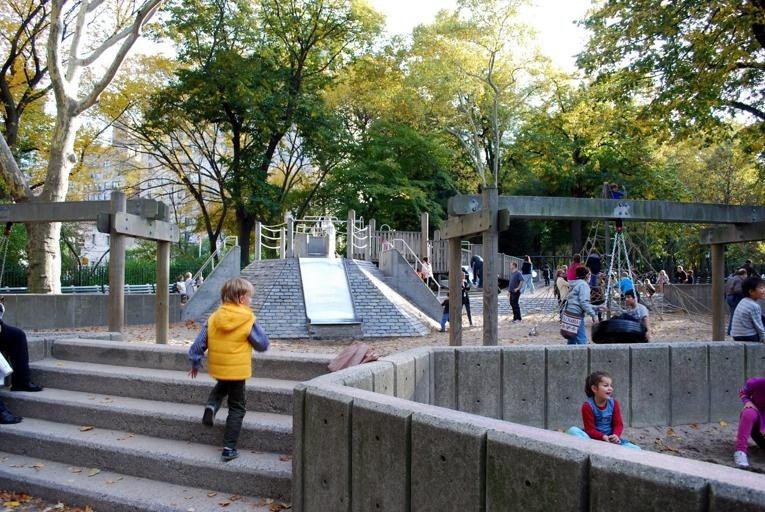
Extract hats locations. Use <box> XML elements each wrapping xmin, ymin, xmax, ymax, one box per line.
<box><xmin>576</xmin><ymin>267</ymin><xmax>589</xmax><ymax>279</ymax></box>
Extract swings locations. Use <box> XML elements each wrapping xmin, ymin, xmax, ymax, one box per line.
<box><xmin>591</xmin><ymin>226</ymin><xmax>645</xmax><ymax>344</ymax></box>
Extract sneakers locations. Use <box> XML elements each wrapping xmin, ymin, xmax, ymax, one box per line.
<box><xmin>222</xmin><ymin>448</ymin><xmax>239</xmax><ymax>459</ymax></box>
<box><xmin>733</xmin><ymin>450</ymin><xmax>750</xmax><ymax>468</ymax></box>
<box><xmin>203</xmin><ymin>401</ymin><xmax>217</xmax><ymax>426</ymax></box>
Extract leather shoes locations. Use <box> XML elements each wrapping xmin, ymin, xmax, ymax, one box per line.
<box><xmin>12</xmin><ymin>381</ymin><xmax>43</xmax><ymax>391</ymax></box>
<box><xmin>0</xmin><ymin>401</ymin><xmax>22</xmax><ymax>424</ymax></box>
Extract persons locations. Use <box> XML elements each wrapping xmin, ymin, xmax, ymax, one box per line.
<box><xmin>0</xmin><ymin>351</ymin><xmax>24</xmax><ymax>426</ymax></box>
<box><xmin>542</xmin><ymin>264</ymin><xmax>550</xmax><ymax>287</ymax></box>
<box><xmin>565</xmin><ymin>371</ymin><xmax>642</xmax><ymax>451</ymax></box>
<box><xmin>177</xmin><ymin>272</ymin><xmax>204</xmax><ymax>302</ymax></box>
<box><xmin>606</xmin><ymin>179</ymin><xmax>626</xmax><ymax>232</ymax></box>
<box><xmin>522</xmin><ymin>254</ymin><xmax>534</xmax><ymax>294</ymax></box>
<box><xmin>0</xmin><ymin>298</ymin><xmax>43</xmax><ymax>392</ymax></box>
<box><xmin>553</xmin><ymin>252</ymin><xmax>765</xmax><ymax>344</ymax></box>
<box><xmin>732</xmin><ymin>375</ymin><xmax>764</xmax><ymax>471</ymax></box>
<box><xmin>508</xmin><ymin>262</ymin><xmax>524</xmax><ymax>323</ymax></box>
<box><xmin>187</xmin><ymin>277</ymin><xmax>270</xmax><ymax>461</ymax></box>
<box><xmin>415</xmin><ymin>253</ymin><xmax>484</xmax><ymax>330</ymax></box>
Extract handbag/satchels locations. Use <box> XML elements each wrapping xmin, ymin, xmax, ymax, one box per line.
<box><xmin>560</xmin><ymin>310</ymin><xmax>583</xmax><ymax>339</ymax></box>
